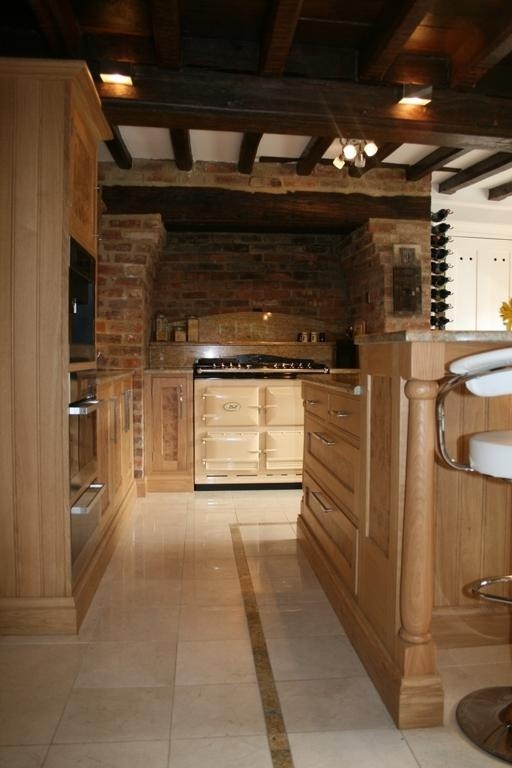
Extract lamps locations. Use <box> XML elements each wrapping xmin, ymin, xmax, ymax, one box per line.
<box><xmin>333</xmin><ymin>137</ymin><xmax>379</xmax><ymax>170</ymax></box>
<box><xmin>99</xmin><ymin>61</ymin><xmax>134</xmax><ymax>89</ymax></box>
<box><xmin>395</xmin><ymin>83</ymin><xmax>433</xmax><ymax>106</ymax></box>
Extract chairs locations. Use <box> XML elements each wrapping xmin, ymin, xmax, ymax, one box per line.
<box><xmin>439</xmin><ymin>345</ymin><xmax>512</xmax><ymax>762</ymax></box>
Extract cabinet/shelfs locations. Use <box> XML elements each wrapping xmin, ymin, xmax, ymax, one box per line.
<box><xmin>147</xmin><ymin>375</ymin><xmax>195</xmax><ymax>493</ymax></box>
<box><xmin>96</xmin><ymin>375</ymin><xmax>134</xmax><ymax>514</ymax></box>
<box><xmin>298</xmin><ymin>375</ymin><xmax>370</xmax><ymax>601</ymax></box>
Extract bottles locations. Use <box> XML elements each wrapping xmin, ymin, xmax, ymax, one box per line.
<box><xmin>154</xmin><ymin>314</ymin><xmax>199</xmax><ymax>342</ymax></box>
<box><xmin>430</xmin><ymin>207</ymin><xmax>452</xmax><ymax>328</ymax></box>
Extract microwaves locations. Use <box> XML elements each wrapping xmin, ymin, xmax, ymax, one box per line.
<box><xmin>69</xmin><ymin>238</ymin><xmax>95</xmax><ymax>343</ymax></box>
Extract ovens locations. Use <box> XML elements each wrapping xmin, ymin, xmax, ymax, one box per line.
<box><xmin>70</xmin><ymin>369</ymin><xmax>107</xmax><ymax>561</ymax></box>
<box><xmin>195</xmin><ymin>379</ymin><xmax>305</xmax><ymax>486</ymax></box>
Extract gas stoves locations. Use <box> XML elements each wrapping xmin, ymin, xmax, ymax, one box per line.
<box><xmin>196</xmin><ymin>355</ymin><xmax>317</xmax><ymax>371</ymax></box>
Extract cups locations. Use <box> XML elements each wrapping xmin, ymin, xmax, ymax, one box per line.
<box><xmin>300</xmin><ymin>331</ymin><xmax>325</xmax><ymax>342</ymax></box>
